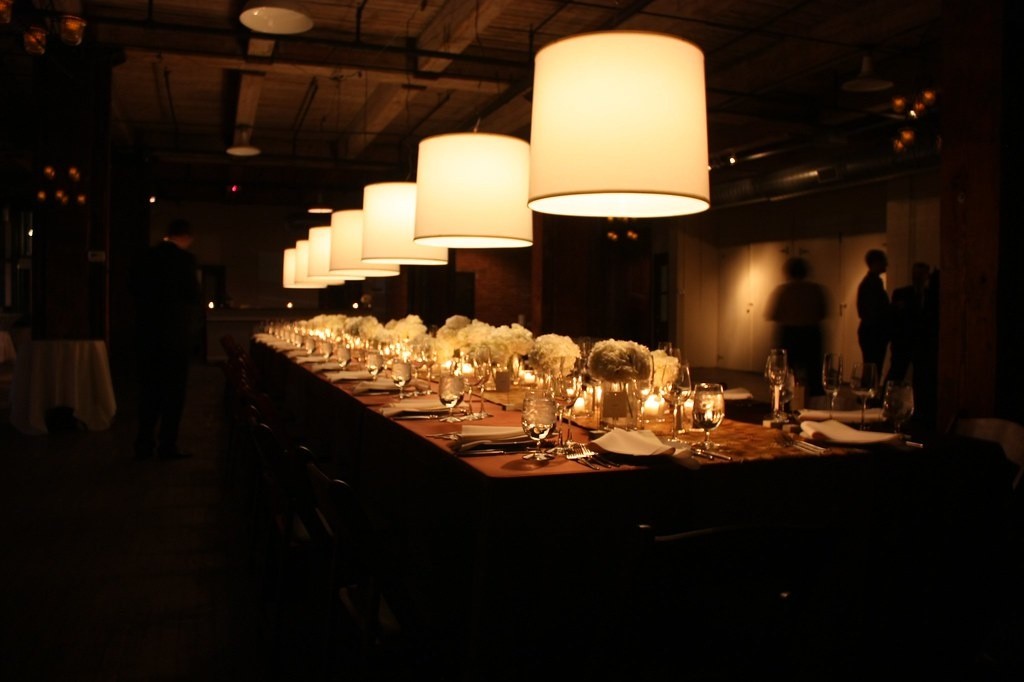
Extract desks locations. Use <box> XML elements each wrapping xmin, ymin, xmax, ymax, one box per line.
<box><xmin>9</xmin><ymin>339</ymin><xmax>118</xmax><ymax>437</ymax></box>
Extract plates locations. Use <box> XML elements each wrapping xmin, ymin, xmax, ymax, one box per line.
<box><xmin>469</xmin><ymin>439</ymin><xmax>535</xmax><ymax>447</ymax></box>
<box><xmin>800</xmin><ymin>431</ymin><xmax>810</xmax><ymax>439</ymax></box>
<box><xmin>370</xmin><ymin>386</ymin><xmax>401</xmax><ymax>391</ymax></box>
<box><xmin>406</xmin><ymin>409</ymin><xmax>450</xmax><ymax>414</ymax></box>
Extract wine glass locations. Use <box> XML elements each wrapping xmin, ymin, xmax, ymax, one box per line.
<box><xmin>850</xmin><ymin>363</ymin><xmax>880</xmax><ymax>431</ymax></box>
<box><xmin>261</xmin><ymin>317</ymin><xmax>334</xmax><ymax>366</ymax></box>
<box><xmin>885</xmin><ymin>383</ymin><xmax>914</xmax><ymax>434</ymax></box>
<box><xmin>332</xmin><ymin>319</ymin><xmax>392</xmax><ymax>381</ymax></box>
<box><xmin>439</xmin><ymin>346</ymin><xmax>491</xmax><ymax>423</ymax></box>
<box><xmin>569</xmin><ymin>337</ymin><xmax>604</xmax><ymax>395</ymax></box>
<box><xmin>661</xmin><ymin>365</ymin><xmax>692</xmax><ymax>443</ymax></box>
<box><xmin>522</xmin><ymin>356</ymin><xmax>582</xmax><ymax>460</ymax></box>
<box><xmin>391</xmin><ymin>344</ymin><xmax>436</xmax><ymax>399</ymax></box>
<box><xmin>765</xmin><ymin>349</ymin><xmax>795</xmax><ymax>422</ymax></box>
<box><xmin>823</xmin><ymin>352</ymin><xmax>842</xmax><ymax>421</ymax></box>
<box><xmin>694</xmin><ymin>383</ymin><xmax>725</xmax><ymax>453</ymax></box>
<box><xmin>658</xmin><ymin>342</ymin><xmax>681</xmax><ymax>364</ymax></box>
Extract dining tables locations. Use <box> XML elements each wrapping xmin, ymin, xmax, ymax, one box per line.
<box><xmin>250</xmin><ymin>334</ymin><xmax>933</xmax><ymax>682</ymax></box>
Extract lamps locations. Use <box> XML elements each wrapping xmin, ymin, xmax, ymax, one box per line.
<box><xmin>413</xmin><ymin>0</ymin><xmax>533</xmax><ymax>248</ymax></box>
<box><xmin>283</xmin><ymin>248</ymin><xmax>327</xmax><ymax>288</ymax></box>
<box><xmin>526</xmin><ymin>29</ymin><xmax>712</xmax><ymax>219</ymax></box>
<box><xmin>307</xmin><ymin>191</ymin><xmax>334</xmax><ymax>214</ymax></box>
<box><xmin>226</xmin><ymin>126</ymin><xmax>262</xmax><ymax>157</ymax></box>
<box><xmin>294</xmin><ymin>239</ymin><xmax>344</xmax><ymax>285</ymax></box>
<box><xmin>360</xmin><ymin>20</ymin><xmax>449</xmax><ymax>265</ymax></box>
<box><xmin>307</xmin><ymin>226</ymin><xmax>366</xmax><ymax>280</ymax></box>
<box><xmin>329</xmin><ymin>67</ymin><xmax>400</xmax><ymax>277</ymax></box>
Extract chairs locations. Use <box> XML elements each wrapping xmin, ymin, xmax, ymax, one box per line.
<box><xmin>218</xmin><ymin>334</ymin><xmax>471</xmax><ymax>682</ymax></box>
<box><xmin>944</xmin><ymin>417</ymin><xmax>1024</xmax><ymax>491</ymax></box>
<box><xmin>787</xmin><ymin>379</ymin><xmax>870</xmax><ymax>423</ymax></box>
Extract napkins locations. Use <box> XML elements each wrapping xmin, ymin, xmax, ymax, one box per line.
<box><xmin>629</xmin><ymin>430</ymin><xmax>694</xmax><ymax>461</ymax></box>
<box><xmin>286</xmin><ymin>350</ymin><xmax>308</xmax><ymax>358</ymax></box>
<box><xmin>311</xmin><ymin>362</ymin><xmax>343</xmax><ymax>373</ymax></box>
<box><xmin>296</xmin><ymin>355</ymin><xmax>326</xmax><ymax>364</ymax></box>
<box><xmin>324</xmin><ymin>370</ymin><xmax>377</xmax><ymax>382</ymax></box>
<box><xmin>378</xmin><ymin>398</ymin><xmax>468</xmax><ymax>418</ymax></box>
<box><xmin>456</xmin><ymin>425</ymin><xmax>530</xmax><ymax>449</ymax></box>
<box><xmin>800</xmin><ymin>418</ymin><xmax>904</xmax><ymax>446</ymax></box>
<box><xmin>794</xmin><ymin>407</ymin><xmax>884</xmax><ymax>422</ymax></box>
<box><xmin>352</xmin><ymin>377</ymin><xmax>418</xmax><ymax>395</ymax></box>
<box><xmin>585</xmin><ymin>427</ymin><xmax>676</xmax><ymax>456</ymax></box>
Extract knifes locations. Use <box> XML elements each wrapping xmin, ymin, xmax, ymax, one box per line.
<box><xmin>669</xmin><ymin>438</ymin><xmax>731</xmax><ymax>461</ymax></box>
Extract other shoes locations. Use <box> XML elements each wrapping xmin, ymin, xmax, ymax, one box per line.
<box><xmin>157</xmin><ymin>448</ymin><xmax>193</xmax><ymax>459</ymax></box>
<box><xmin>135</xmin><ymin>452</ymin><xmax>159</xmax><ymax>462</ymax></box>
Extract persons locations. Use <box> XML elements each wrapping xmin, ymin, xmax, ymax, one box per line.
<box><xmin>856</xmin><ymin>250</ymin><xmax>907</xmax><ymax>388</ymax></box>
<box><xmin>882</xmin><ymin>262</ymin><xmax>940</xmax><ymax>434</ymax></box>
<box><xmin>128</xmin><ymin>218</ymin><xmax>203</xmax><ymax>459</ymax></box>
<box><xmin>771</xmin><ymin>256</ymin><xmax>828</xmax><ymax>397</ymax></box>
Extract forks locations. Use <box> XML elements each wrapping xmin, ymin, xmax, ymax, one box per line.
<box><xmin>775</xmin><ymin>432</ymin><xmax>831</xmax><ymax>455</ymax></box>
<box><xmin>565</xmin><ymin>444</ymin><xmax>619</xmax><ymax>469</ymax></box>
